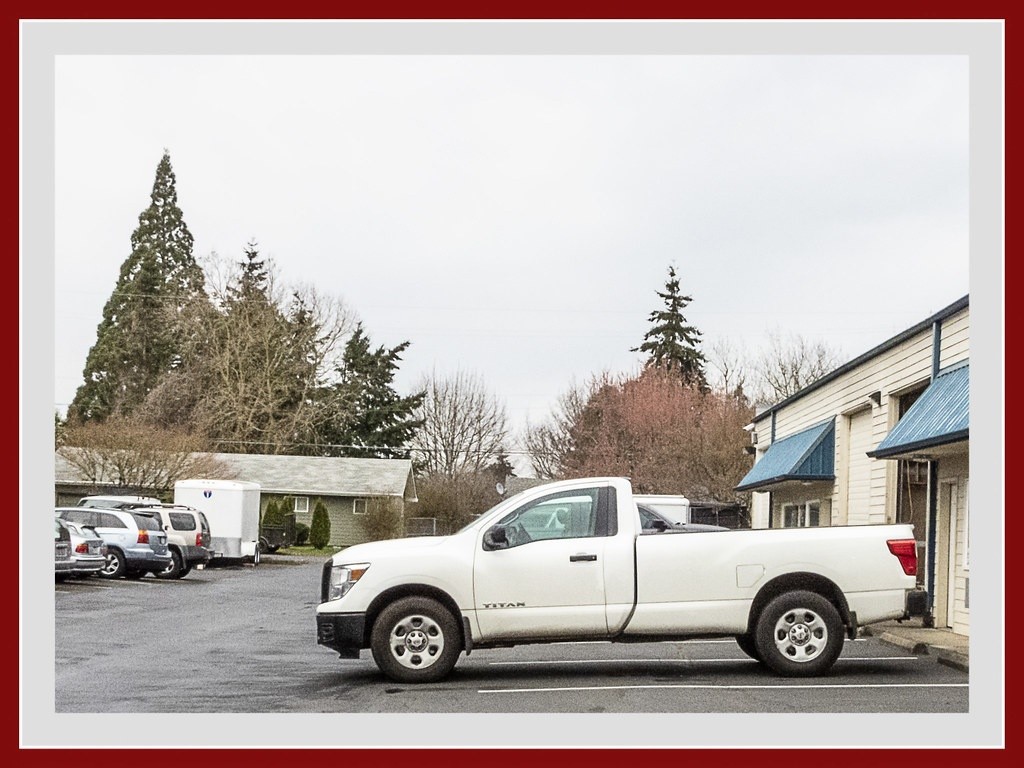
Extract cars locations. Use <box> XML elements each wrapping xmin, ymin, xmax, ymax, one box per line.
<box><xmin>55</xmin><ymin>517</ymin><xmax>105</xmax><ymax>583</ymax></box>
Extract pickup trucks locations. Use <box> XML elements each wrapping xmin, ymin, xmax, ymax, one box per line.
<box><xmin>316</xmin><ymin>477</ymin><xmax>918</xmax><ymax>685</ymax></box>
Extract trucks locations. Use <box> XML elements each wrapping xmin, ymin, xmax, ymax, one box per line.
<box><xmin>74</xmin><ymin>479</ymin><xmax>261</xmax><ymax>566</ymax></box>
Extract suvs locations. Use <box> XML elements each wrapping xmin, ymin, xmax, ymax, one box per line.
<box><xmin>54</xmin><ymin>506</ymin><xmax>172</xmax><ymax>580</ymax></box>
<box><xmin>112</xmin><ymin>506</ymin><xmax>214</xmax><ymax>579</ymax></box>
